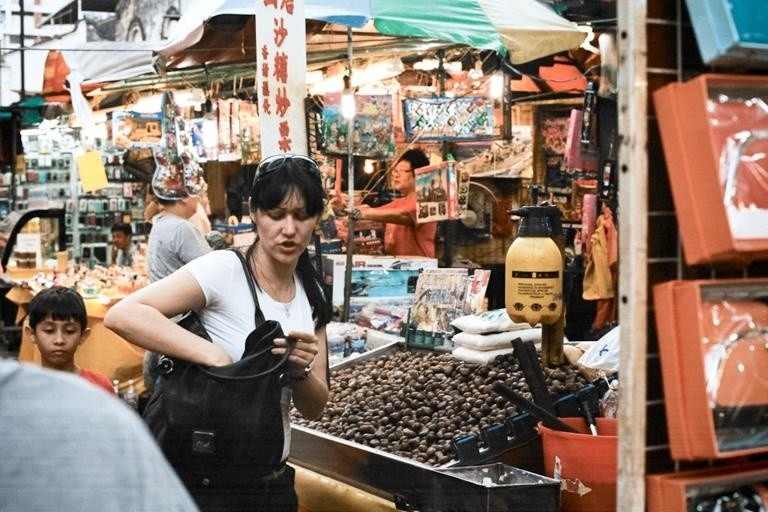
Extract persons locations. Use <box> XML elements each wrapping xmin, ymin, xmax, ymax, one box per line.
<box><xmin>143</xmin><ymin>173</ymin><xmax>215</xmax><ymax>391</ymax></box>
<box><xmin>1</xmin><ymin>354</ymin><xmax>201</xmax><ymax>511</ymax></box>
<box><xmin>24</xmin><ymin>286</ymin><xmax>117</xmax><ymax>396</ymax></box>
<box><xmin>111</xmin><ymin>221</ymin><xmax>137</xmax><ymax>267</ymax></box>
<box><xmin>103</xmin><ymin>154</ymin><xmax>336</xmax><ymax>512</ymax></box>
<box><xmin>353</xmin><ymin>147</ymin><xmax>438</xmax><ymax>257</ymax></box>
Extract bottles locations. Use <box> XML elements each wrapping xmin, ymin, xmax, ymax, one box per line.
<box><xmin>401</xmin><ymin>325</ymin><xmax>454</xmax><ymax>346</ymax></box>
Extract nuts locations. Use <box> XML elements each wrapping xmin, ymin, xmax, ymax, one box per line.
<box><xmin>289</xmin><ymin>348</ymin><xmax>588</xmax><ymax>466</ymax></box>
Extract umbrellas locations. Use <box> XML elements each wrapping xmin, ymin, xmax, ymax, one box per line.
<box><xmin>155</xmin><ymin>1</ymin><xmax>589</xmax><ymax>323</ymax></box>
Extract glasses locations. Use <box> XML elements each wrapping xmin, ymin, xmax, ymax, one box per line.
<box><xmin>391</xmin><ymin>169</ymin><xmax>414</xmax><ymax>175</ymax></box>
<box><xmin>257</xmin><ymin>153</ymin><xmax>324</xmax><ymax>177</ymax></box>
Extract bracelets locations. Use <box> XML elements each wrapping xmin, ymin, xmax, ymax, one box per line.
<box><xmin>292</xmin><ymin>367</ymin><xmax>312</xmax><ymax>383</ymax></box>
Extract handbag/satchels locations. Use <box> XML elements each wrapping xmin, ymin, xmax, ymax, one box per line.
<box><xmin>135</xmin><ymin>313</ymin><xmax>296</xmax><ymax>493</ymax></box>
